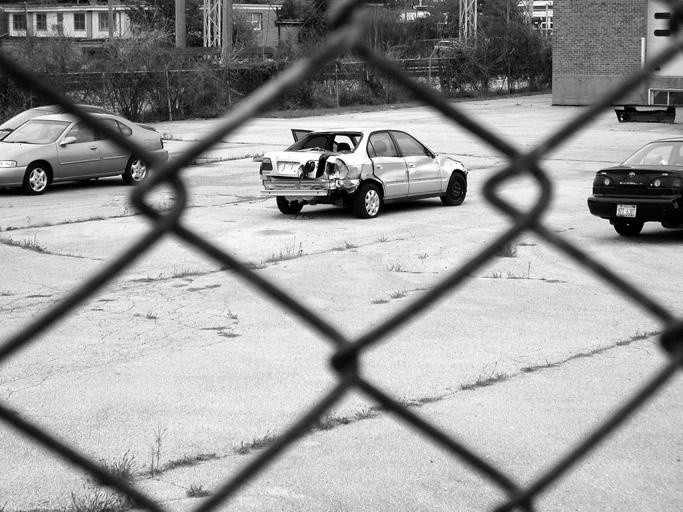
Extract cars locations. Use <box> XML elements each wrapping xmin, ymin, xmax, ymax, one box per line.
<box><xmin>0</xmin><ymin>113</ymin><xmax>172</xmax><ymax>198</ymax></box>
<box><xmin>0</xmin><ymin>101</ymin><xmax>155</xmax><ymax>139</ymax></box>
<box><xmin>433</xmin><ymin>41</ymin><xmax>450</xmax><ymax>51</ymax></box>
<box><xmin>585</xmin><ymin>134</ymin><xmax>683</xmax><ymax>237</ymax></box>
<box><xmin>251</xmin><ymin>126</ymin><xmax>471</xmax><ymax>219</ymax></box>
